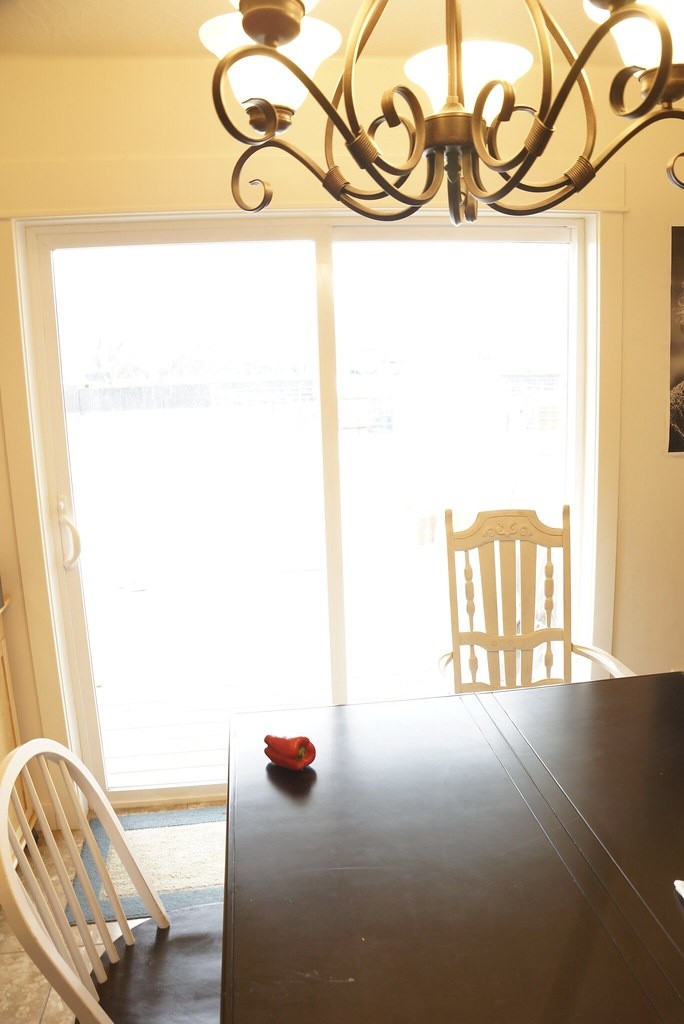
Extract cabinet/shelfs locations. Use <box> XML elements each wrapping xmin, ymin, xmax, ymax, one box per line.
<box><xmin>1</xmin><ymin>600</ymin><xmax>40</xmax><ymax>883</ymax></box>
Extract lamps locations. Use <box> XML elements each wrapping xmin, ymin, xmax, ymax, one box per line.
<box><xmin>197</xmin><ymin>1</ymin><xmax>684</xmax><ymax>225</ymax></box>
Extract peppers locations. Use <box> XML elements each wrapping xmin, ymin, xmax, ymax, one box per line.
<box><xmin>263</xmin><ymin>733</ymin><xmax>316</xmax><ymax>770</ymax></box>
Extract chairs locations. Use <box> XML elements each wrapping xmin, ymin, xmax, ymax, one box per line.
<box><xmin>0</xmin><ymin>737</ymin><xmax>222</xmax><ymax>1023</ymax></box>
<box><xmin>429</xmin><ymin>506</ymin><xmax>636</xmax><ymax>698</ymax></box>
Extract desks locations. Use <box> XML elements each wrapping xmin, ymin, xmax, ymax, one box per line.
<box><xmin>221</xmin><ymin>672</ymin><xmax>683</xmax><ymax>1024</ymax></box>
